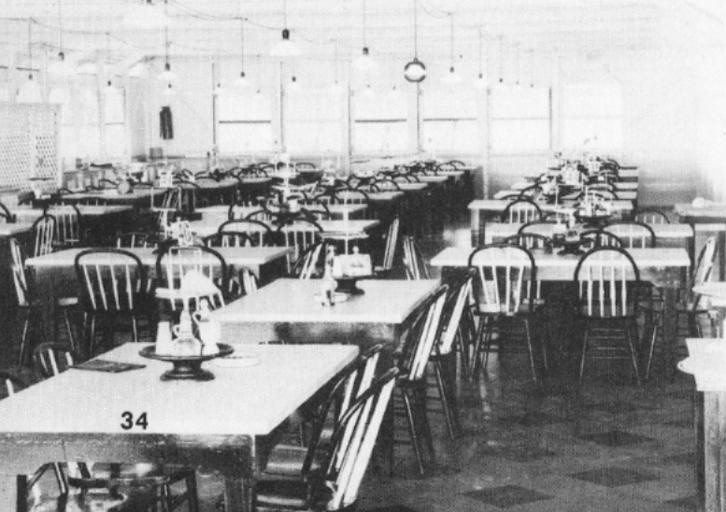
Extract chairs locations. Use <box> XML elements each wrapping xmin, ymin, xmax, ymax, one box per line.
<box><xmin>348</xmin><ymin>286</ymin><xmax>450</xmax><ymax>476</ymax></box>
<box><xmin>154</xmin><ymin>244</ymin><xmax>228</xmax><ymax>317</ymax></box>
<box><xmin>7</xmin><ymin>236</ymin><xmax>81</xmax><ymax>373</ymax></box>
<box><xmin>645</xmin><ymin>235</ymin><xmax>719</xmax><ymax>383</ymax></box>
<box><xmin>75</xmin><ymin>249</ymin><xmax>150</xmax><ymax>353</ymax></box>
<box><xmin>0</xmin><ymin>156</ymin><xmax>726</xmax><ymax>324</ymax></box>
<box><xmin>32</xmin><ymin>342</ymin><xmax>200</xmax><ymax>512</ymax></box>
<box><xmin>16</xmin><ymin>0</ymin><xmax>533</xmax><ymax>94</ymax></box>
<box><xmin>3</xmin><ymin>376</ymin><xmax>147</xmax><ymax>512</ymax></box>
<box><xmin>251</xmin><ymin>370</ymin><xmax>402</xmax><ymax>512</ymax></box>
<box><xmin>572</xmin><ymin>248</ymin><xmax>643</xmax><ymax>385</ymax></box>
<box><xmin>467</xmin><ymin>243</ymin><xmax>550</xmax><ymax>380</ymax></box>
<box><xmin>258</xmin><ymin>345</ymin><xmax>387</xmax><ymax>478</ymax></box>
<box><xmin>389</xmin><ymin>270</ymin><xmax>477</xmax><ymax>440</ymax></box>
<box><xmin>181</xmin><ymin>268</ymin><xmax>226</xmax><ymax>341</ymax></box>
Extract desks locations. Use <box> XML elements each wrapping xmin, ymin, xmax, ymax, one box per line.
<box><xmin>430</xmin><ymin>245</ymin><xmax>686</xmax><ymax>381</ymax></box>
<box><xmin>2</xmin><ymin>343</ymin><xmax>360</xmax><ymax>512</ymax></box>
<box><xmin>207</xmin><ymin>277</ymin><xmax>440</xmax><ymax>472</ymax></box>
<box><xmin>678</xmin><ymin>339</ymin><xmax>726</xmax><ymax>512</ymax></box>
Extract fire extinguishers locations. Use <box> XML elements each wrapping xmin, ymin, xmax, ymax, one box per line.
<box><xmin>159</xmin><ymin>106</ymin><xmax>173</xmax><ymax>139</ymax></box>
